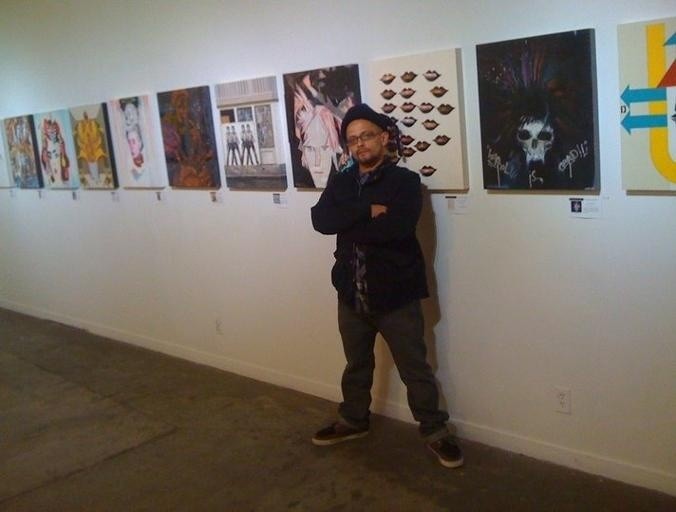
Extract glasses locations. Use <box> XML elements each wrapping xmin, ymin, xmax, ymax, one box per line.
<box><xmin>345</xmin><ymin>132</ymin><xmax>374</xmax><ymax>146</ymax></box>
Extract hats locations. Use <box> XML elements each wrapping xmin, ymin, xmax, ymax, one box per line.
<box><xmin>341</xmin><ymin>104</ymin><xmax>385</xmax><ymax>138</ymax></box>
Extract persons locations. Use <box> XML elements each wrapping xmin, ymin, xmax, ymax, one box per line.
<box><xmin>309</xmin><ymin>103</ymin><xmax>464</xmax><ymax>469</ymax></box>
<box><xmin>40</xmin><ymin>119</ymin><xmax>70</xmax><ymax>186</ymax></box>
<box><xmin>126</xmin><ymin>123</ymin><xmax>145</xmax><ymax>170</ymax></box>
<box><xmin>226</xmin><ymin>123</ymin><xmax>259</xmax><ymax>167</ymax></box>
<box><xmin>294</xmin><ymin>84</ymin><xmax>355</xmax><ymax>187</ymax></box>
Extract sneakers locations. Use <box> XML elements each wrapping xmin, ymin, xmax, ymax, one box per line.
<box><xmin>428</xmin><ymin>434</ymin><xmax>464</xmax><ymax>468</ymax></box>
<box><xmin>312</xmin><ymin>421</ymin><xmax>368</xmax><ymax>446</ymax></box>
